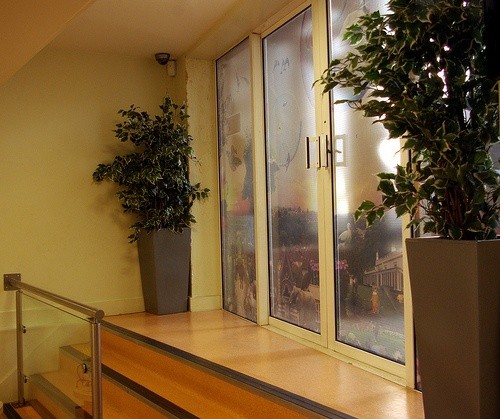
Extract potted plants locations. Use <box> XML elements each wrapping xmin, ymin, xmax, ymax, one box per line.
<box><xmin>90</xmin><ymin>87</ymin><xmax>210</xmax><ymax>315</ymax></box>
<box><xmin>309</xmin><ymin>0</ymin><xmax>500</xmax><ymax>419</ymax></box>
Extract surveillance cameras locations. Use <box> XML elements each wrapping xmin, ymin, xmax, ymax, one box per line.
<box><xmin>155</xmin><ymin>53</ymin><xmax>169</xmax><ymax>65</ymax></box>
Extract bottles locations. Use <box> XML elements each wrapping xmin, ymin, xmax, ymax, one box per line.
<box><xmin>370</xmin><ymin>291</ymin><xmax>378</xmax><ymax>314</ymax></box>
